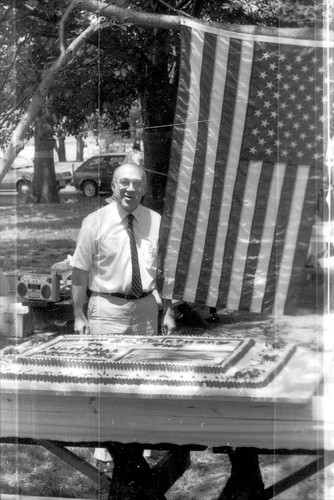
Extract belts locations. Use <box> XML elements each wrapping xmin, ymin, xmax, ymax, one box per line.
<box><xmin>99</xmin><ymin>289</ymin><xmax>154</xmax><ymax>301</ymax></box>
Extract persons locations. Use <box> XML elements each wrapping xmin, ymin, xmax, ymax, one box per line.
<box><xmin>71</xmin><ymin>162</ymin><xmax>178</xmax><ymax>334</ymax></box>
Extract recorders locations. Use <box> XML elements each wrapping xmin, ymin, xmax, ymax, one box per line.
<box><xmin>16</xmin><ymin>270</ymin><xmax>61</xmax><ymax>302</ymax></box>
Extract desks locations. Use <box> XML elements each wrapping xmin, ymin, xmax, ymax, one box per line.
<box><xmin>0</xmin><ymin>437</ymin><xmax>334</xmax><ymax>500</ymax></box>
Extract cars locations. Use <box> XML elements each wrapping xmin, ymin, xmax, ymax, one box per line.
<box><xmin>72</xmin><ymin>154</ymin><xmax>141</xmax><ymax>197</ymax></box>
<box><xmin>2</xmin><ymin>153</ymin><xmax>72</xmax><ymax>196</ymax></box>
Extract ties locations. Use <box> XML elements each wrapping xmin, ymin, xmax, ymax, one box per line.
<box><xmin>125</xmin><ymin>212</ymin><xmax>144</xmax><ymax>299</ymax></box>
